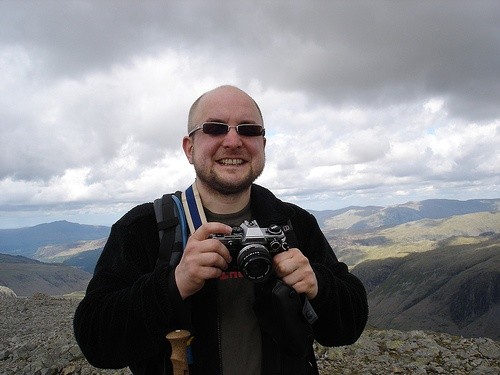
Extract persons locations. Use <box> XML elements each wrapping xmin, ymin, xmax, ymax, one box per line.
<box><xmin>73</xmin><ymin>85</ymin><xmax>368</xmax><ymax>375</ymax></box>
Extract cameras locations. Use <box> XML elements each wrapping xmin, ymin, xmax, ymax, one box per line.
<box><xmin>206</xmin><ymin>220</ymin><xmax>290</xmax><ymax>281</ymax></box>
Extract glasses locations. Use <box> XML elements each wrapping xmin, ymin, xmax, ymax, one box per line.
<box><xmin>188</xmin><ymin>122</ymin><xmax>266</xmax><ymax>137</ymax></box>
